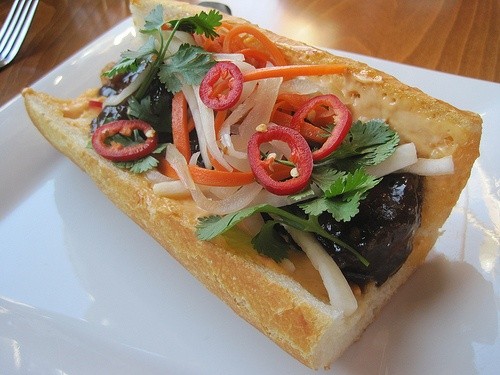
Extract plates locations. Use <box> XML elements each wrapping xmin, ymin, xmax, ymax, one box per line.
<box><xmin>0</xmin><ymin>16</ymin><xmax>500</xmax><ymax>375</ymax></box>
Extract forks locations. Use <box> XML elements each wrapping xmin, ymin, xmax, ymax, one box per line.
<box><xmin>0</xmin><ymin>0</ymin><xmax>40</xmax><ymax>69</ymax></box>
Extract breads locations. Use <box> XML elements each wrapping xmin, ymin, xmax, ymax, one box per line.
<box><xmin>19</xmin><ymin>0</ymin><xmax>480</xmax><ymax>369</ymax></box>
